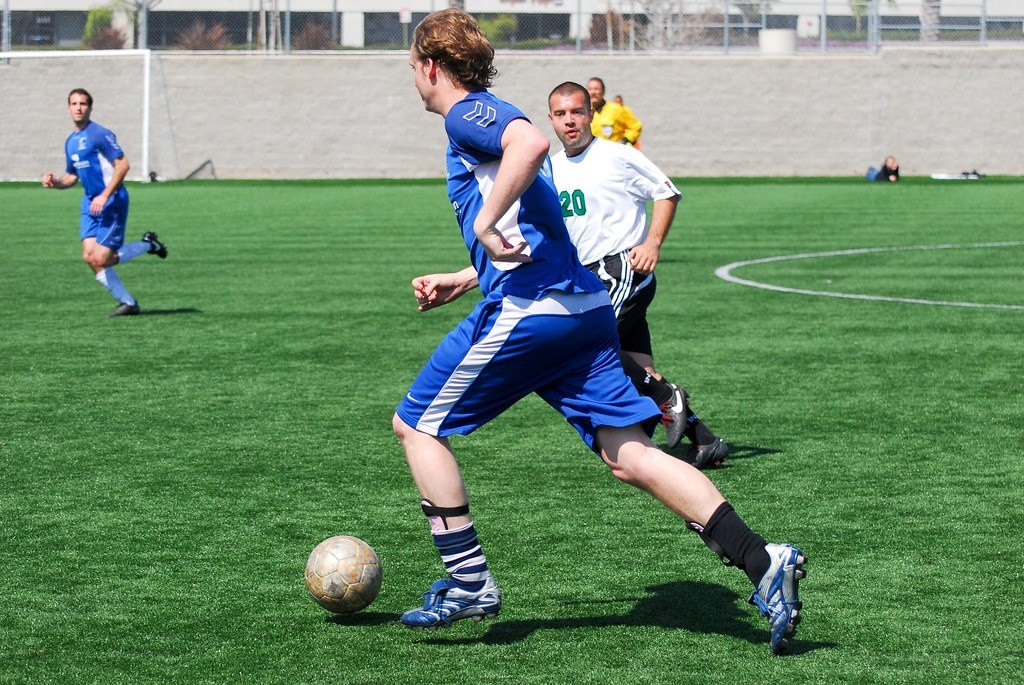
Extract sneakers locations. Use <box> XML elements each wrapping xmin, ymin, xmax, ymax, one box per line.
<box><xmin>684</xmin><ymin>437</ymin><xmax>729</xmax><ymax>471</ymax></box>
<box><xmin>659</xmin><ymin>383</ymin><xmax>690</xmax><ymax>449</ymax></box>
<box><xmin>401</xmin><ymin>576</ymin><xmax>501</xmax><ymax>631</ymax></box>
<box><xmin>106</xmin><ymin>300</ymin><xmax>140</xmax><ymax>317</ymax></box>
<box><xmin>747</xmin><ymin>542</ymin><xmax>807</xmax><ymax>654</ymax></box>
<box><xmin>142</xmin><ymin>231</ymin><xmax>167</xmax><ymax>258</ymax></box>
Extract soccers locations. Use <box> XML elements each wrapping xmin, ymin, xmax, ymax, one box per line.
<box><xmin>302</xmin><ymin>534</ymin><xmax>383</xmax><ymax>613</ymax></box>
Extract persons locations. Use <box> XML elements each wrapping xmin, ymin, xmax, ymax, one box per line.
<box><xmin>547</xmin><ymin>79</ymin><xmax>730</xmax><ymax>470</ymax></box>
<box><xmin>864</xmin><ymin>157</ymin><xmax>899</xmax><ymax>183</ymax></box>
<box><xmin>42</xmin><ymin>88</ymin><xmax>168</xmax><ymax>318</ymax></box>
<box><xmin>391</xmin><ymin>10</ymin><xmax>806</xmax><ymax>658</ymax></box>
<box><xmin>586</xmin><ymin>77</ymin><xmax>642</xmax><ymax>150</ymax></box>
